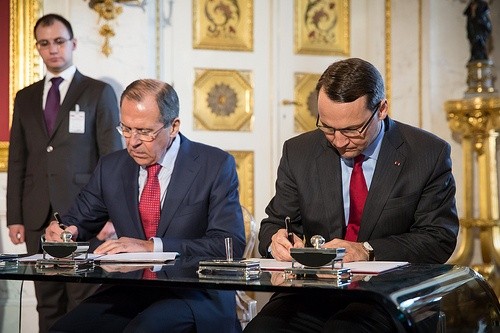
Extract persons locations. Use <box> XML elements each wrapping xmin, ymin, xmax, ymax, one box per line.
<box><xmin>6</xmin><ymin>13</ymin><xmax>123</xmax><ymax>333</ymax></box>
<box><xmin>243</xmin><ymin>58</ymin><xmax>460</xmax><ymax>333</ymax></box>
<box><xmin>41</xmin><ymin>78</ymin><xmax>247</xmax><ymax>333</ymax></box>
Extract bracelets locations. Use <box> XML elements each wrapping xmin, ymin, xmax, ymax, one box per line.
<box><xmin>268</xmin><ymin>244</ymin><xmax>275</xmax><ymax>257</ymax></box>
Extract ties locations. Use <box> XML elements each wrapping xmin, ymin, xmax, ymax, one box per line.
<box><xmin>139</xmin><ymin>164</ymin><xmax>162</xmax><ymax>279</ymax></box>
<box><xmin>344</xmin><ymin>154</ymin><xmax>368</xmax><ymax>242</ymax></box>
<box><xmin>43</xmin><ymin>77</ymin><xmax>64</xmax><ymax>136</ymax></box>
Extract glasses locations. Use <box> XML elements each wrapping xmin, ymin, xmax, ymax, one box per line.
<box><xmin>316</xmin><ymin>106</ymin><xmax>378</xmax><ymax>138</ymax></box>
<box><xmin>35</xmin><ymin>36</ymin><xmax>72</xmax><ymax>50</ymax></box>
<box><xmin>116</xmin><ymin>121</ymin><xmax>170</xmax><ymax>142</ymax></box>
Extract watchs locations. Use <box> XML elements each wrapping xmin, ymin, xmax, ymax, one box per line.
<box><xmin>361</xmin><ymin>240</ymin><xmax>374</xmax><ymax>261</ymax></box>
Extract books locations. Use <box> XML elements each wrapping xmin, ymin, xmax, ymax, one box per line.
<box><xmin>240</xmin><ymin>258</ymin><xmax>411</xmax><ymax>274</ymax></box>
<box><xmin>11</xmin><ymin>251</ymin><xmax>180</xmax><ymax>263</ymax></box>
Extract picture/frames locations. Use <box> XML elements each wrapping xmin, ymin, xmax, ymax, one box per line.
<box><xmin>192</xmin><ymin>0</ymin><xmax>255</xmax><ymax>51</ymax></box>
<box><xmin>194</xmin><ymin>66</ymin><xmax>255</xmax><ymax>133</ymax></box>
<box><xmin>291</xmin><ymin>0</ymin><xmax>352</xmax><ymax>58</ymax></box>
<box><xmin>224</xmin><ymin>149</ymin><xmax>255</xmax><ymax>319</ymax></box>
<box><xmin>294</xmin><ymin>71</ymin><xmax>321</xmax><ymax>133</ymax></box>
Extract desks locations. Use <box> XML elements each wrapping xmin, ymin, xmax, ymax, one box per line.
<box><xmin>0</xmin><ymin>258</ymin><xmax>500</xmax><ymax>333</ymax></box>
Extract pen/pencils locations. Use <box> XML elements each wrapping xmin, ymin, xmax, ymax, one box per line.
<box><xmin>54</xmin><ymin>211</ymin><xmax>66</xmax><ymax>231</ymax></box>
<box><xmin>285</xmin><ymin>216</ymin><xmax>294</xmax><ymax>246</ymax></box>
